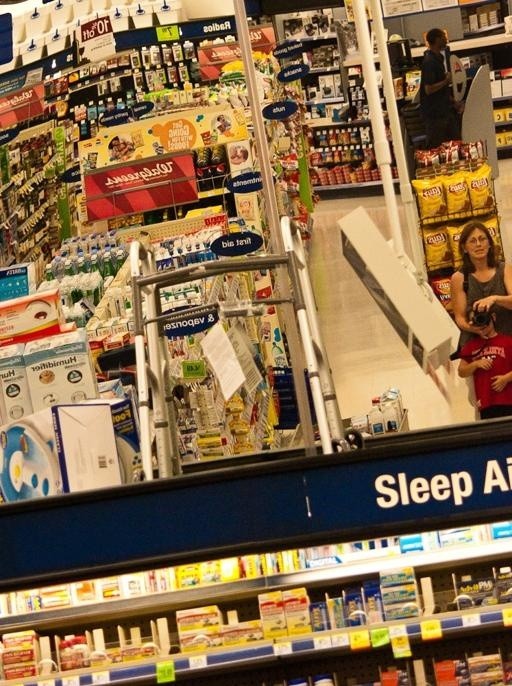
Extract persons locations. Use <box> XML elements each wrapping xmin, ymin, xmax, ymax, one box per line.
<box><xmin>419</xmin><ymin>27</ymin><xmax>461</xmax><ymax>148</ymax></box>
<box><xmin>451</xmin><ymin>221</ymin><xmax>512</xmax><ymax>347</ymax></box>
<box><xmin>457</xmin><ymin>305</ymin><xmax>512</xmax><ymax>419</ymax></box>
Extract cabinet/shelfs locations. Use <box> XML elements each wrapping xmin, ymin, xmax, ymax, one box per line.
<box><xmin>2</xmin><ymin>1</ymin><xmax>511</xmax><ymax>684</ymax></box>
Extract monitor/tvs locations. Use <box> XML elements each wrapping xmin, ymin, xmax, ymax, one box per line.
<box><xmin>387</xmin><ymin>39</ymin><xmax>412</xmax><ymax>66</ymax></box>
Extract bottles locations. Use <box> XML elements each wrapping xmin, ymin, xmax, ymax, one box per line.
<box><xmin>368</xmin><ymin>404</ymin><xmax>398</xmax><ymax>434</ymax></box>
<box><xmin>45</xmin><ymin>230</ymin><xmax>126</xmax><ymax>326</ymax></box>
